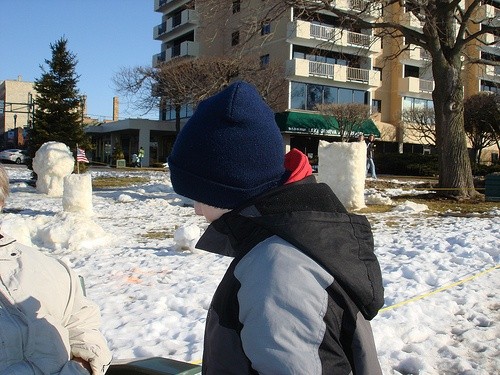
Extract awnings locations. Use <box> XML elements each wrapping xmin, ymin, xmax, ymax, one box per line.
<box><xmin>274</xmin><ymin>112</ymin><xmax>380</xmax><ymax>138</ymax></box>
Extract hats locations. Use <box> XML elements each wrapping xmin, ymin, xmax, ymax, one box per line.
<box><xmin>168</xmin><ymin>82</ymin><xmax>291</xmax><ymax>209</ymax></box>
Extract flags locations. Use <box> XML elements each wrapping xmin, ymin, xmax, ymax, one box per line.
<box><xmin>77</xmin><ymin>148</ymin><xmax>89</xmax><ymax>163</ymax></box>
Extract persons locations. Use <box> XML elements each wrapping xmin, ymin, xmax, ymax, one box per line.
<box><xmin>132</xmin><ymin>153</ymin><xmax>138</xmax><ymax>166</ymax></box>
<box><xmin>167</xmin><ymin>81</ymin><xmax>385</xmax><ymax>375</ymax></box>
<box><xmin>0</xmin><ymin>163</ymin><xmax>113</xmax><ymax>375</ymax></box>
<box><xmin>139</xmin><ymin>147</ymin><xmax>144</xmax><ymax>168</ymax></box>
<box><xmin>357</xmin><ymin>134</ymin><xmax>376</xmax><ymax>178</ymax></box>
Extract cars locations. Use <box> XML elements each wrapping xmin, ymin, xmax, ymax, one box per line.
<box><xmin>124</xmin><ymin>152</ymin><xmax>138</xmax><ymax>167</ymax></box>
<box><xmin>0</xmin><ymin>148</ymin><xmax>29</xmax><ymax>165</ymax></box>
